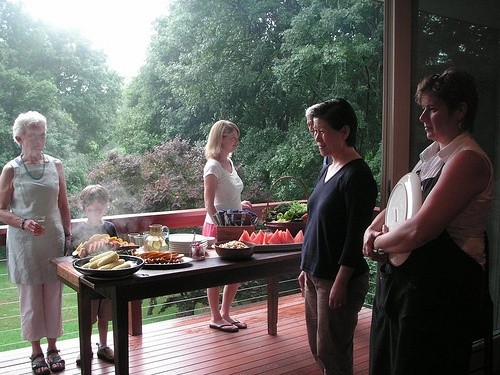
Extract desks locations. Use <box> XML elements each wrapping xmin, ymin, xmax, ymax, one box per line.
<box><xmin>49</xmin><ymin>250</ymin><xmax>303</xmax><ymax>375</ymax></box>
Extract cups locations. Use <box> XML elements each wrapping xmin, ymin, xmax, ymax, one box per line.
<box><xmin>207</xmin><ymin>237</ymin><xmax>215</xmax><ymax>248</ymax></box>
<box><xmin>35</xmin><ymin>216</ymin><xmax>46</xmax><ymax>232</ymax></box>
<box><xmin>128</xmin><ymin>231</ymin><xmax>149</xmax><ymax>247</ymax></box>
<box><xmin>192</xmin><ymin>243</ymin><xmax>206</xmax><ymax>261</ymax></box>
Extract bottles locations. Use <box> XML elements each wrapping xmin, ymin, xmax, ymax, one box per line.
<box><xmin>213</xmin><ymin>209</ymin><xmax>257</xmax><ymax>226</ymax></box>
<box><xmin>147</xmin><ymin>224</ymin><xmax>169</xmax><ymax>250</ymax></box>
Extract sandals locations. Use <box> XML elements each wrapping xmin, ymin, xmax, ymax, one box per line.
<box><xmin>47</xmin><ymin>350</ymin><xmax>65</xmax><ymax>373</ymax></box>
<box><xmin>30</xmin><ymin>352</ymin><xmax>50</xmax><ymax>375</ymax></box>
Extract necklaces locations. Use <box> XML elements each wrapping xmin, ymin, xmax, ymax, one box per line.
<box><xmin>21</xmin><ymin>154</ymin><xmax>45</xmax><ymax>180</ymax></box>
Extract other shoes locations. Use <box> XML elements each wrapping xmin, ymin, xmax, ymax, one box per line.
<box><xmin>76</xmin><ymin>352</ymin><xmax>93</xmax><ymax>364</ymax></box>
<box><xmin>96</xmin><ymin>343</ymin><xmax>114</xmax><ymax>363</ymax></box>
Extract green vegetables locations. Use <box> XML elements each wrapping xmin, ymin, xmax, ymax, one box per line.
<box><xmin>269</xmin><ymin>200</ymin><xmax>307</xmax><ymax>218</ymax></box>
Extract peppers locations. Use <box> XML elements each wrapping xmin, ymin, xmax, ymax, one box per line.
<box><xmin>277</xmin><ymin>213</ymin><xmax>284</xmax><ymax>220</ymax></box>
<box><xmin>284</xmin><ymin>211</ymin><xmax>293</xmax><ymax>219</ymax></box>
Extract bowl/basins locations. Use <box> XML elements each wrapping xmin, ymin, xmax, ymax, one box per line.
<box><xmin>74</xmin><ymin>255</ymin><xmax>144</xmax><ymax>280</ymax></box>
<box><xmin>215</xmin><ymin>241</ymin><xmax>256</xmax><ymax>260</ymax></box>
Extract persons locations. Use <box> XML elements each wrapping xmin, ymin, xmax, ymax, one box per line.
<box><xmin>362</xmin><ymin>65</ymin><xmax>495</xmax><ymax>375</ymax></box>
<box><xmin>202</xmin><ymin>120</ymin><xmax>250</xmax><ymax>333</ymax></box>
<box><xmin>298</xmin><ymin>98</ymin><xmax>378</xmax><ymax>375</ymax></box>
<box><xmin>0</xmin><ymin>110</ymin><xmax>71</xmax><ymax>375</ymax></box>
<box><xmin>71</xmin><ymin>185</ymin><xmax>118</xmax><ymax>364</ymax></box>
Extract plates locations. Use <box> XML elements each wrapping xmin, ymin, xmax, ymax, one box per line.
<box><xmin>143</xmin><ymin>256</ymin><xmax>194</xmax><ymax>266</ymax></box>
<box><xmin>169</xmin><ymin>234</ymin><xmax>208</xmax><ymax>255</ymax></box>
<box><xmin>384</xmin><ymin>172</ymin><xmax>422</xmax><ymax>266</ymax></box>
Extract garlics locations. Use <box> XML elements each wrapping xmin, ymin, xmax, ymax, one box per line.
<box><xmin>219</xmin><ymin>240</ymin><xmax>249</xmax><ymax>248</ymax></box>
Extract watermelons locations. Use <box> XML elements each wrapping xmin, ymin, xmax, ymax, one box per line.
<box><xmin>239</xmin><ymin>228</ymin><xmax>304</xmax><ymax>244</ymax></box>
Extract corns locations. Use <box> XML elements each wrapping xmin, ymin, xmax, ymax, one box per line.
<box><xmin>81</xmin><ymin>251</ymin><xmax>131</xmax><ymax>271</ymax></box>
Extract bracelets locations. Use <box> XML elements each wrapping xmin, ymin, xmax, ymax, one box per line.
<box><xmin>66</xmin><ymin>234</ymin><xmax>72</xmax><ymax>236</ymax></box>
<box><xmin>22</xmin><ymin>219</ymin><xmax>27</xmax><ymax>230</ymax></box>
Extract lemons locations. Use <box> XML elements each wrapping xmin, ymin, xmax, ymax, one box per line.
<box><xmin>152</xmin><ymin>240</ymin><xmax>162</xmax><ymax>249</ymax></box>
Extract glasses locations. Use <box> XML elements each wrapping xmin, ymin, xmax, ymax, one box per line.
<box><xmin>439</xmin><ymin>70</ymin><xmax>454</xmax><ymax>88</ymax></box>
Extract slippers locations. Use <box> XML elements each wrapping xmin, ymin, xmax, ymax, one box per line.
<box><xmin>231</xmin><ymin>319</ymin><xmax>247</xmax><ymax>329</ymax></box>
<box><xmin>210</xmin><ymin>323</ymin><xmax>239</xmax><ymax>333</ymax></box>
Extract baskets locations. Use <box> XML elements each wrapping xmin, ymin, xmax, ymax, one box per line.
<box><xmin>218</xmin><ymin>225</ymin><xmax>255</xmax><ymax>242</ymax></box>
<box><xmin>263</xmin><ymin>177</ymin><xmax>309</xmax><ymax>239</ymax></box>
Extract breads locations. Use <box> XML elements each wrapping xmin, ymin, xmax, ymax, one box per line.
<box><xmin>72</xmin><ymin>233</ymin><xmax>137</xmax><ymax>257</ymax></box>
<box><xmin>136</xmin><ymin>251</ymin><xmax>185</xmax><ymax>260</ymax></box>
